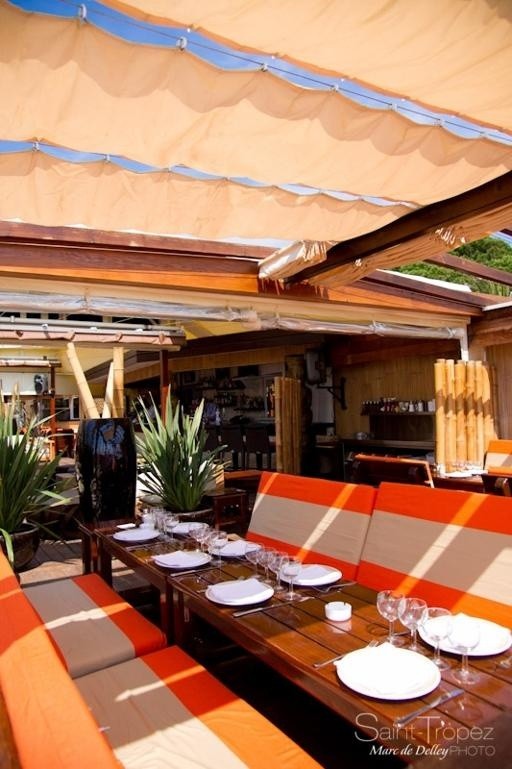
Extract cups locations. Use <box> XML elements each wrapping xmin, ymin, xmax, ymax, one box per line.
<box><xmin>431</xmin><ymin>459</ymin><xmax>483</xmax><ymax>477</ymax></box>
<box><xmin>354</xmin><ymin>432</ymin><xmax>363</xmax><ymax>440</ymax></box>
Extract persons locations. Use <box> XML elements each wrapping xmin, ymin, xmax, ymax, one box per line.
<box><xmin>199</xmin><ymin>396</ymin><xmax>221</xmax><ymax>426</ymax></box>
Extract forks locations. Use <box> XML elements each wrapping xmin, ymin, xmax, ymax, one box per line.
<box><xmin>493</xmin><ymin>657</ymin><xmax>512</xmax><ymax>668</ymax></box>
<box><xmin>312</xmin><ymin>640</ymin><xmax>379</xmax><ymax>668</ymax></box>
<box><xmin>311</xmin><ymin>581</ymin><xmax>357</xmax><ymax>594</ymax></box>
<box><xmin>196</xmin><ymin>575</ymin><xmax>245</xmax><ymax>593</ymax></box>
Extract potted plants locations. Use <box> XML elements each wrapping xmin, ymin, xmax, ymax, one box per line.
<box><xmin>131</xmin><ymin>381</ymin><xmax>235</xmax><ymax>520</ymax></box>
<box><xmin>0</xmin><ymin>381</ymin><xmax>94</xmax><ymax>571</ymax></box>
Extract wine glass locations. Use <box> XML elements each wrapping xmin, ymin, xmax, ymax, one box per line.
<box><xmin>376</xmin><ymin>588</ymin><xmax>479</xmax><ymax>685</ymax></box>
<box><xmin>111</xmin><ymin>506</ymin><xmax>260</xmax><ymax>570</ymax></box>
<box><xmin>245</xmin><ymin>543</ymin><xmax>301</xmax><ymax>600</ymax></box>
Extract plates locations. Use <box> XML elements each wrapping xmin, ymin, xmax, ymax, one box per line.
<box><xmin>417</xmin><ymin>614</ymin><xmax>512</xmax><ymax>657</ymax></box>
<box><xmin>206</xmin><ymin>580</ymin><xmax>273</xmax><ymax>608</ymax></box>
<box><xmin>278</xmin><ymin>564</ymin><xmax>342</xmax><ymax>588</ymax></box>
<box><xmin>336</xmin><ymin>647</ymin><xmax>442</xmax><ymax>702</ymax></box>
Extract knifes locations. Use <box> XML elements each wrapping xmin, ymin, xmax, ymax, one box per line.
<box><xmin>170</xmin><ymin>566</ymin><xmax>217</xmax><ymax>578</ymax></box>
<box><xmin>391</xmin><ymin>689</ymin><xmax>463</xmax><ymax>727</ymax></box>
<box><xmin>232</xmin><ymin>594</ymin><xmax>314</xmax><ymax>619</ymax></box>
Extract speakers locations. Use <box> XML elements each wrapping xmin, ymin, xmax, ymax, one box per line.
<box><xmin>34</xmin><ymin>374</ymin><xmax>48</xmax><ymax>395</ymax></box>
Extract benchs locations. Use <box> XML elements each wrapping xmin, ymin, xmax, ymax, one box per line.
<box><xmin>1</xmin><ymin>418</ymin><xmax>512</xmax><ymax>769</ymax></box>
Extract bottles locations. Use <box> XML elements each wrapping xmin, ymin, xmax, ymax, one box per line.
<box><xmin>359</xmin><ymin>397</ymin><xmax>435</xmax><ymax>411</ymax></box>
<box><xmin>214</xmin><ymin>407</ymin><xmax>221</xmax><ymax>426</ymax></box>
<box><xmin>231</xmin><ymin>448</ymin><xmax>238</xmax><ymax>469</ymax></box>
<box><xmin>241</xmin><ymin>435</ymin><xmax>248</xmax><ymax>470</ymax></box>
<box><xmin>215</xmin><ymin>392</ymin><xmax>259</xmax><ymax>409</ymax></box>
<box><xmin>217</xmin><ymin>433</ymin><xmax>223</xmax><ymax>464</ymax></box>
